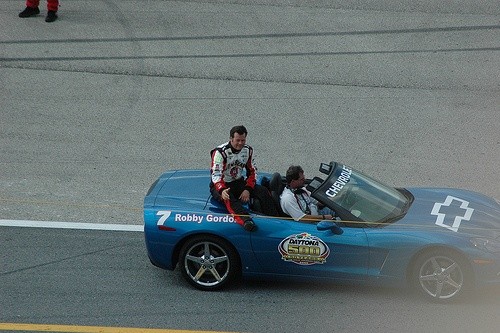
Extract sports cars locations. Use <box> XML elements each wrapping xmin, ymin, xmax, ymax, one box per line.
<box><xmin>142</xmin><ymin>161</ymin><xmax>500</xmax><ymax>304</ymax></box>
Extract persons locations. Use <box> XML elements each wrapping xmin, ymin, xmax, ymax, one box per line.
<box><xmin>279</xmin><ymin>165</ymin><xmax>342</xmax><ymax>221</ymax></box>
<box><xmin>19</xmin><ymin>0</ymin><xmax>59</xmax><ymax>23</ymax></box>
<box><xmin>209</xmin><ymin>125</ymin><xmax>280</xmax><ymax>232</ymax></box>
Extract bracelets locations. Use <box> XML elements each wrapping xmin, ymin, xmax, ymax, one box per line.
<box><xmin>322</xmin><ymin>215</ymin><xmax>325</xmax><ymax>219</ymax></box>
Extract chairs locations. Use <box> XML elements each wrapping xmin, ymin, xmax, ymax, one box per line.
<box><xmin>254</xmin><ymin>186</ymin><xmax>276</xmax><ymax>215</ymax></box>
<box><xmin>270</xmin><ymin>172</ymin><xmax>284</xmax><ymax>210</ymax></box>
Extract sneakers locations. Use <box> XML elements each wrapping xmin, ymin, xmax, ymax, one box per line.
<box><xmin>18</xmin><ymin>6</ymin><xmax>40</xmax><ymax>18</ymax></box>
<box><xmin>243</xmin><ymin>217</ymin><xmax>254</xmax><ymax>230</ymax></box>
<box><xmin>45</xmin><ymin>10</ymin><xmax>58</xmax><ymax>22</ymax></box>
